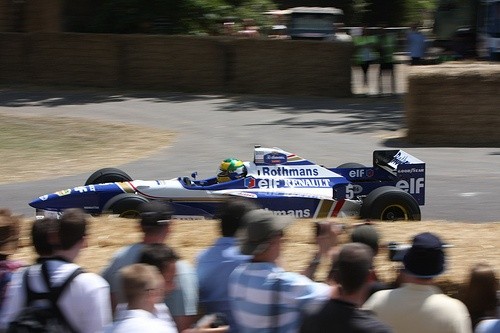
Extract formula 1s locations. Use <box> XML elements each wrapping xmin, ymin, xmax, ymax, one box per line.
<box><xmin>28</xmin><ymin>145</ymin><xmax>426</xmax><ymax>220</ymax></box>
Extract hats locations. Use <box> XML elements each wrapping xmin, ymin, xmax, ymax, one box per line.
<box><xmin>241</xmin><ymin>209</ymin><xmax>292</xmax><ymax>255</ymax></box>
<box><xmin>404</xmin><ymin>233</ymin><xmax>444</xmax><ymax>277</ymax></box>
<box><xmin>0</xmin><ymin>213</ymin><xmax>19</xmax><ymax>254</ymax></box>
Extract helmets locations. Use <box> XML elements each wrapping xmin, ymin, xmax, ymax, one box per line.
<box><xmin>217</xmin><ymin>159</ymin><xmax>247</xmax><ymax>182</ymax></box>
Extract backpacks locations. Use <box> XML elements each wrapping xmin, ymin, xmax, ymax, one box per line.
<box><xmin>8</xmin><ymin>264</ymin><xmax>86</xmax><ymax>333</ymax></box>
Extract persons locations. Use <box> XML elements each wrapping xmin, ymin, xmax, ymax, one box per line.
<box><xmin>226</xmin><ymin>209</ymin><xmax>341</xmax><ymax>333</ymax></box>
<box><xmin>114</xmin><ymin>247</ymin><xmax>220</xmax><ymax>333</ymax></box>
<box><xmin>101</xmin><ymin>199</ymin><xmax>198</xmax><ymax>333</ymax></box>
<box><xmin>453</xmin><ymin>265</ymin><xmax>500</xmax><ymax>333</ymax></box>
<box><xmin>0</xmin><ymin>206</ymin><xmax>113</xmax><ymax>333</ymax></box>
<box><xmin>353</xmin><ymin>27</ymin><xmax>375</xmax><ymax>88</ymax></box>
<box><xmin>406</xmin><ymin>25</ymin><xmax>425</xmax><ymax>63</ymax></box>
<box><xmin>300</xmin><ymin>218</ymin><xmax>405</xmax><ymax>304</ymax></box>
<box><xmin>183</xmin><ymin>158</ymin><xmax>247</xmax><ymax>187</ymax></box>
<box><xmin>301</xmin><ymin>243</ymin><xmax>394</xmax><ymax>333</ymax></box>
<box><xmin>110</xmin><ymin>263</ymin><xmax>229</xmax><ymax>333</ymax></box>
<box><xmin>374</xmin><ymin>30</ymin><xmax>398</xmax><ymax>94</ymax></box>
<box><xmin>196</xmin><ymin>201</ymin><xmax>255</xmax><ymax>318</ymax></box>
<box><xmin>361</xmin><ymin>233</ymin><xmax>471</xmax><ymax>333</ymax></box>
